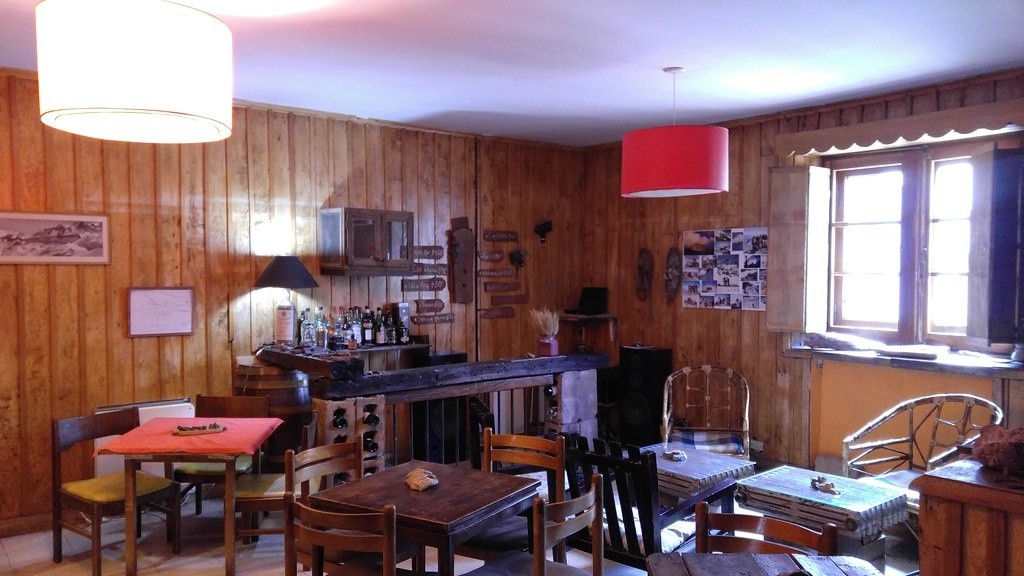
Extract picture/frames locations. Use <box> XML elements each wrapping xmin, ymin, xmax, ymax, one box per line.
<box><xmin>128</xmin><ymin>286</ymin><xmax>196</xmax><ymax>337</ymax></box>
<box><xmin>0</xmin><ymin>211</ymin><xmax>110</xmax><ymax>266</ymax></box>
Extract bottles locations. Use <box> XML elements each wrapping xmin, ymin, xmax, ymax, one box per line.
<box><xmin>296</xmin><ymin>305</ymin><xmax>410</xmax><ymax>350</ymax></box>
<box><xmin>333</xmin><ymin>409</ymin><xmax>382</xmax><ymax>488</ymax></box>
<box><xmin>545</xmin><ymin>386</ymin><xmax>558</xmax><ymax>440</ymax></box>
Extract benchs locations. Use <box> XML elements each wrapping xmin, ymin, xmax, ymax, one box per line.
<box><xmin>544</xmin><ymin>429</ymin><xmax>738</xmax><ymax>571</ymax></box>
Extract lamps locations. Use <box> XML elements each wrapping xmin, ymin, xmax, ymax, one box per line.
<box><xmin>36</xmin><ymin>0</ymin><xmax>231</xmax><ymax>144</ymax></box>
<box><xmin>254</xmin><ymin>255</ymin><xmax>320</xmax><ymax>306</ymax></box>
<box><xmin>621</xmin><ymin>67</ymin><xmax>728</xmax><ymax>198</ymax></box>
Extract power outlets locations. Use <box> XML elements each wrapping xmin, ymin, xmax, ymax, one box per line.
<box><xmin>751</xmin><ymin>441</ymin><xmax>764</xmax><ymax>451</ymax></box>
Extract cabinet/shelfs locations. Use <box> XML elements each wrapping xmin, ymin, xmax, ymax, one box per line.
<box><xmin>317</xmin><ymin>210</ymin><xmax>414</xmax><ymax>278</ymax></box>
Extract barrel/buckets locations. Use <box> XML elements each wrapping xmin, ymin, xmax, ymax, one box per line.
<box><xmin>235</xmin><ymin>360</ymin><xmax>311</xmax><ymax>475</ymax></box>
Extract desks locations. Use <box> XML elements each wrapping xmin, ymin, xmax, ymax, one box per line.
<box><xmin>617</xmin><ymin>442</ymin><xmax>756</xmax><ymax>503</ymax></box>
<box><xmin>307</xmin><ymin>460</ymin><xmax>542</xmax><ymax>576</ymax></box>
<box><xmin>736</xmin><ymin>466</ymin><xmax>908</xmax><ymax>574</ymax></box>
<box><xmin>909</xmin><ymin>457</ymin><xmax>1024</xmax><ymax>576</ymax></box>
<box><xmin>90</xmin><ymin>417</ymin><xmax>284</xmax><ymax>576</ymax></box>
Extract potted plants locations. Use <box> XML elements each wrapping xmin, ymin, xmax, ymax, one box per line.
<box><xmin>530</xmin><ymin>309</ymin><xmax>559</xmax><ymax>357</ymax></box>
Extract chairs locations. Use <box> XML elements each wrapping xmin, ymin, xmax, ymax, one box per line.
<box><xmin>663</xmin><ymin>364</ymin><xmax>751</xmax><ymax>463</ymax></box>
<box><xmin>841</xmin><ymin>392</ymin><xmax>1003</xmax><ymax>575</ymax></box>
<box><xmin>285</xmin><ymin>434</ymin><xmax>426</xmax><ymax>576</ymax></box>
<box><xmin>453</xmin><ymin>474</ymin><xmax>605</xmax><ymax>576</ymax></box>
<box><xmin>232</xmin><ymin>410</ymin><xmax>320</xmax><ymax>539</ymax></box>
<box><xmin>52</xmin><ymin>407</ymin><xmax>181</xmax><ymax>576</ymax></box>
<box><xmin>695</xmin><ymin>502</ymin><xmax>836</xmax><ymax>559</ymax></box>
<box><xmin>284</xmin><ymin>491</ymin><xmax>397</xmax><ymax>576</ymax></box>
<box><xmin>174</xmin><ymin>395</ymin><xmax>267</xmax><ymax>554</ymax></box>
<box><xmin>454</xmin><ymin>429</ymin><xmax>567</xmax><ymax>564</ymax></box>
